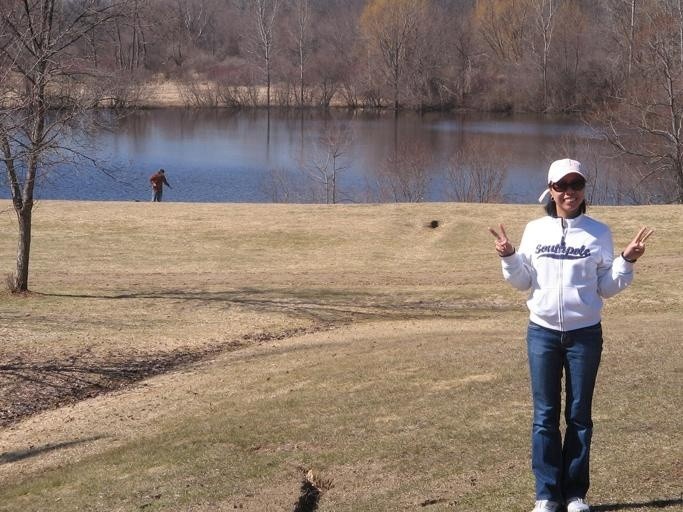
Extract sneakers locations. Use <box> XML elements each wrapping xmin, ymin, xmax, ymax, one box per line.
<box><xmin>532</xmin><ymin>499</ymin><xmax>559</xmax><ymax>512</ymax></box>
<box><xmin>566</xmin><ymin>496</ymin><xmax>590</xmax><ymax>511</ymax></box>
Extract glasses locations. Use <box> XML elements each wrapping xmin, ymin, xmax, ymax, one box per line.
<box><xmin>550</xmin><ymin>179</ymin><xmax>586</xmax><ymax>192</ymax></box>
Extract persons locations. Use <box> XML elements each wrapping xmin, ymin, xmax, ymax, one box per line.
<box><xmin>148</xmin><ymin>168</ymin><xmax>172</xmax><ymax>202</ymax></box>
<box><xmin>486</xmin><ymin>157</ymin><xmax>655</xmax><ymax>512</ymax></box>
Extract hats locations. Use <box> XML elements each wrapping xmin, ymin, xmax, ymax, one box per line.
<box><xmin>547</xmin><ymin>158</ymin><xmax>587</xmax><ymax>186</ymax></box>
